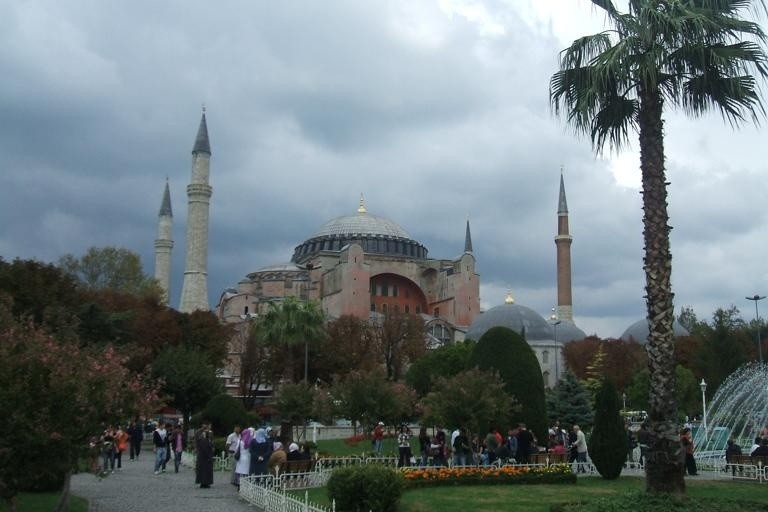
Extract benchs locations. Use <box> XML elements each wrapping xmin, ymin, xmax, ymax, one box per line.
<box><xmin>728</xmin><ymin>455</ymin><xmax>768</xmax><ymax>481</ymax></box>
<box><xmin>527</xmin><ymin>453</ymin><xmax>568</xmax><ymax>467</ymax></box>
<box><xmin>270</xmin><ymin>459</ymin><xmax>313</xmax><ymax>479</ymax></box>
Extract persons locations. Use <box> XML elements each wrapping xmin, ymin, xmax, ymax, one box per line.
<box><xmin>99</xmin><ymin>417</ymin><xmax>317</xmax><ymax>490</ymax></box>
<box><xmin>395</xmin><ymin>417</ymin><xmax>591</xmax><ymax>473</ymax></box>
<box><xmin>747</xmin><ymin>426</ymin><xmax>767</xmax><ymax>476</ymax></box>
<box><xmin>723</xmin><ymin>439</ymin><xmax>742</xmax><ymax>480</ymax></box>
<box><xmin>623</xmin><ymin>422</ymin><xmax>636</xmax><ymax>470</ymax></box>
<box><xmin>683</xmin><ymin>427</ymin><xmax>698</xmax><ymax>476</ymax></box>
<box><xmin>371</xmin><ymin>421</ymin><xmax>385</xmax><ymax>457</ymax></box>
<box><xmin>635</xmin><ymin>423</ymin><xmax>648</xmax><ymax>469</ymax></box>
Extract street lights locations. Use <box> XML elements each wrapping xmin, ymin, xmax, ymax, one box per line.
<box><xmin>745</xmin><ymin>294</ymin><xmax>767</xmax><ymax>365</ymax></box>
<box><xmin>622</xmin><ymin>391</ymin><xmax>627</xmax><ymax>413</ymax></box>
<box><xmin>551</xmin><ymin>320</ymin><xmax>562</xmax><ymax>379</ymax></box>
<box><xmin>699</xmin><ymin>377</ymin><xmax>709</xmax><ymax>451</ymax></box>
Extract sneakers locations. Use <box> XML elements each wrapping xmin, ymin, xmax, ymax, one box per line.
<box><xmin>162</xmin><ymin>468</ymin><xmax>167</xmax><ymax>473</ymax></box>
<box><xmin>154</xmin><ymin>470</ymin><xmax>161</xmax><ymax>475</ymax></box>
<box><xmin>129</xmin><ymin>458</ymin><xmax>135</xmax><ymax>462</ymax></box>
<box><xmin>103</xmin><ymin>470</ymin><xmax>109</xmax><ymax>474</ymax></box>
<box><xmin>136</xmin><ymin>455</ymin><xmax>140</xmax><ymax>461</ymax></box>
<box><xmin>116</xmin><ymin>467</ymin><xmax>124</xmax><ymax>471</ymax></box>
<box><xmin>111</xmin><ymin>469</ymin><xmax>115</xmax><ymax>473</ymax></box>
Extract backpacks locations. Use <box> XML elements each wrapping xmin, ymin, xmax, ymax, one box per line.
<box><xmin>102</xmin><ymin>437</ymin><xmax>115</xmax><ymax>454</ymax></box>
<box><xmin>189</xmin><ymin>430</ymin><xmax>203</xmax><ymax>456</ymax></box>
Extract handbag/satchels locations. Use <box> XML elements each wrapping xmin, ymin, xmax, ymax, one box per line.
<box><xmin>118</xmin><ymin>431</ymin><xmax>129</xmax><ymax>452</ymax></box>
<box><xmin>233</xmin><ymin>438</ymin><xmax>242</xmax><ymax>461</ymax></box>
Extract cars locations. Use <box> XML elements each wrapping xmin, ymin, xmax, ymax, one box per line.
<box><xmin>144</xmin><ymin>413</ymin><xmax>192</xmax><ymax>434</ymax></box>
<box><xmin>304</xmin><ymin>416</ymin><xmax>386</xmax><ymax>428</ymax></box>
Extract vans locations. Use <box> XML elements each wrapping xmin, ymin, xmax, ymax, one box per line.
<box><xmin>621</xmin><ymin>410</ymin><xmax>649</xmax><ymax>420</ymax></box>
